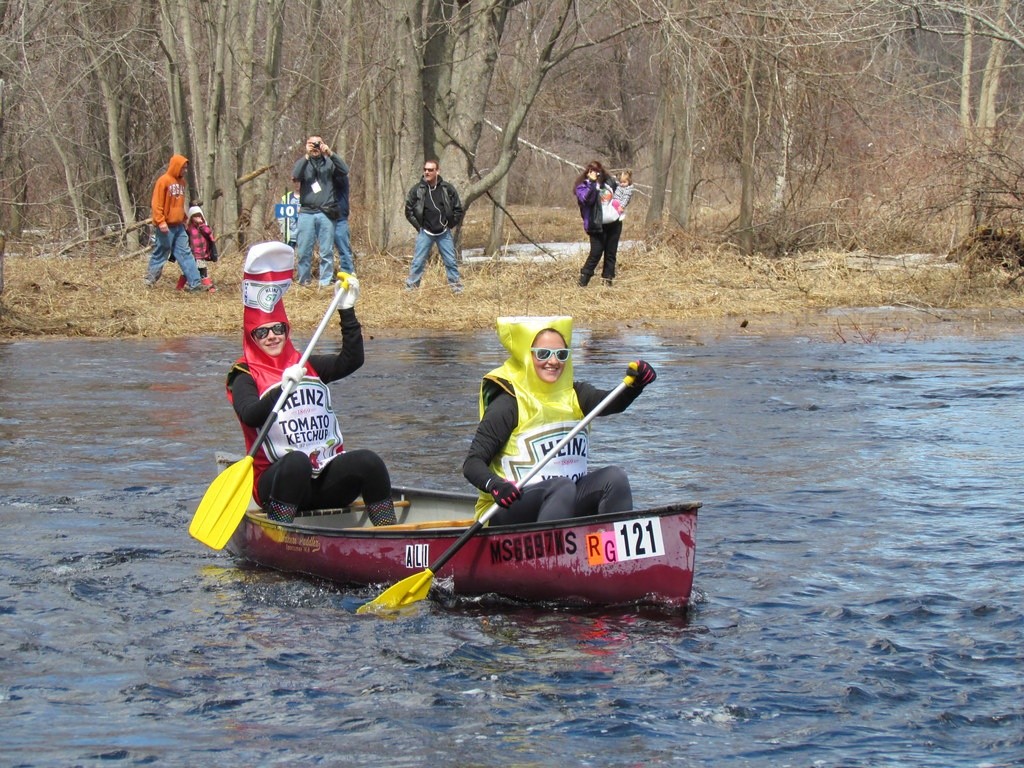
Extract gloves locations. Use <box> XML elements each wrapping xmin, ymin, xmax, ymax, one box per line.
<box><xmin>280</xmin><ymin>364</ymin><xmax>308</xmax><ymax>395</ymax></box>
<box><xmin>333</xmin><ymin>271</ymin><xmax>360</xmax><ymax>310</ymax></box>
<box><xmin>627</xmin><ymin>360</ymin><xmax>657</xmax><ymax>386</ymax></box>
<box><xmin>491</xmin><ymin>479</ymin><xmax>524</xmax><ymax>509</ymax></box>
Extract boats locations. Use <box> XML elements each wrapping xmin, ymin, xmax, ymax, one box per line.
<box><xmin>215</xmin><ymin>449</ymin><xmax>703</xmax><ymax>617</ymax></box>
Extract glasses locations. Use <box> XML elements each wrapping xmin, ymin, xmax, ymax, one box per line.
<box><xmin>529</xmin><ymin>347</ymin><xmax>571</xmax><ymax>362</ymax></box>
<box><xmin>182</xmin><ymin>166</ymin><xmax>190</xmax><ymax>170</ymax></box>
<box><xmin>251</xmin><ymin>324</ymin><xmax>285</xmax><ymax>339</ymax></box>
<box><xmin>424</xmin><ymin>168</ymin><xmax>436</xmax><ymax>172</ymax></box>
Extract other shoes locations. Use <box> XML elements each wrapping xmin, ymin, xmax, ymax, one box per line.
<box><xmin>619</xmin><ymin>213</ymin><xmax>626</xmax><ymax>222</ymax></box>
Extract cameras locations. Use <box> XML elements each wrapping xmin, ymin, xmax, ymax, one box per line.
<box><xmin>596</xmin><ymin>172</ymin><xmax>602</xmax><ymax>177</ymax></box>
<box><xmin>312</xmin><ymin>141</ymin><xmax>320</xmax><ymax>148</ymax></box>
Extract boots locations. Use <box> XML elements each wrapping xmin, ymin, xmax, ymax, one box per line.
<box><xmin>202</xmin><ymin>277</ymin><xmax>216</xmax><ymax>293</ymax></box>
<box><xmin>176</xmin><ymin>275</ymin><xmax>188</xmax><ymax>293</ymax></box>
<box><xmin>578</xmin><ymin>273</ymin><xmax>591</xmax><ymax>288</ymax></box>
<box><xmin>603</xmin><ymin>277</ymin><xmax>612</xmax><ymax>287</ymax></box>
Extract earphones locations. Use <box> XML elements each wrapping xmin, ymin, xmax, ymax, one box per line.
<box><xmin>436</xmin><ymin>172</ymin><xmax>439</xmax><ymax>174</ymax></box>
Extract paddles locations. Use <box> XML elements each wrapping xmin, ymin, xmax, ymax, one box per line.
<box><xmin>189</xmin><ymin>271</ymin><xmax>356</xmax><ymax>551</ymax></box>
<box><xmin>356</xmin><ymin>362</ymin><xmax>638</xmax><ymax>615</ymax></box>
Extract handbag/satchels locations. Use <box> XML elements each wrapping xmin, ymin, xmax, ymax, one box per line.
<box><xmin>320</xmin><ymin>203</ymin><xmax>341</xmax><ymax>220</ymax></box>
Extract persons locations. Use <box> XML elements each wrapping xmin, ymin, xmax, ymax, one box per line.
<box><xmin>225</xmin><ymin>241</ymin><xmax>397</xmax><ymax>527</ymax></box>
<box><xmin>404</xmin><ymin>160</ymin><xmax>463</xmax><ymax>291</ymax></box>
<box><xmin>462</xmin><ymin>315</ymin><xmax>657</xmax><ymax>528</ymax></box>
<box><xmin>571</xmin><ymin>161</ymin><xmax>633</xmax><ymax>289</ymax></box>
<box><xmin>280</xmin><ymin>137</ymin><xmax>355</xmax><ymax>287</ymax></box>
<box><xmin>143</xmin><ymin>156</ymin><xmax>219</xmax><ymax>294</ymax></box>
